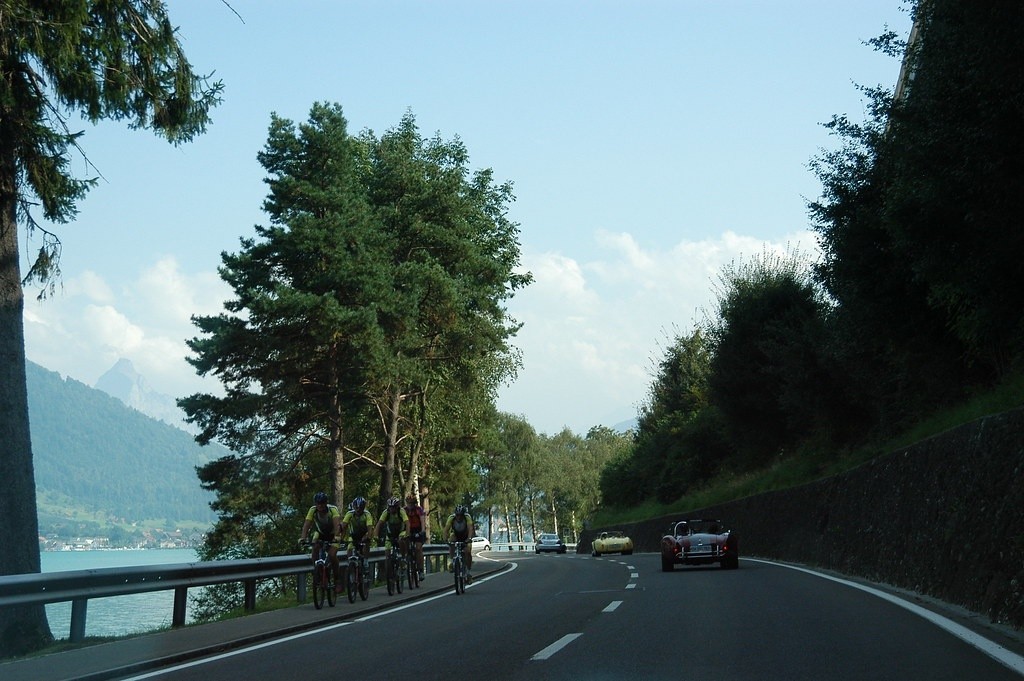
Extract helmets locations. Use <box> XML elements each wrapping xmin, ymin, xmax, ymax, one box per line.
<box><xmin>454</xmin><ymin>504</ymin><xmax>466</xmax><ymax>515</ymax></box>
<box><xmin>406</xmin><ymin>495</ymin><xmax>417</xmax><ymax>503</ymax></box>
<box><xmin>314</xmin><ymin>493</ymin><xmax>328</xmax><ymax>503</ymax></box>
<box><xmin>387</xmin><ymin>497</ymin><xmax>400</xmax><ymax>507</ymax></box>
<box><xmin>352</xmin><ymin>497</ymin><xmax>367</xmax><ymax>506</ymax></box>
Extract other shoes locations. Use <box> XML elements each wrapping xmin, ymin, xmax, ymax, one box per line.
<box><xmin>418</xmin><ymin>568</ymin><xmax>425</xmax><ymax>581</ymax></box>
<box><xmin>335</xmin><ymin>579</ymin><xmax>343</xmax><ymax>593</ymax></box>
<box><xmin>467</xmin><ymin>574</ymin><xmax>473</xmax><ymax>585</ymax></box>
<box><xmin>401</xmin><ymin>558</ymin><xmax>407</xmax><ymax>570</ymax></box>
<box><xmin>449</xmin><ymin>564</ymin><xmax>454</xmax><ymax>573</ymax></box>
<box><xmin>365</xmin><ymin>571</ymin><xmax>370</xmax><ymax>582</ymax></box>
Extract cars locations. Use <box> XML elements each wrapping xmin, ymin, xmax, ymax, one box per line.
<box><xmin>536</xmin><ymin>534</ymin><xmax>561</xmax><ymax>554</ymax></box>
<box><xmin>471</xmin><ymin>537</ymin><xmax>492</xmax><ymax>551</ymax></box>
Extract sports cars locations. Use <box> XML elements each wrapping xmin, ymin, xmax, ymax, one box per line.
<box><xmin>661</xmin><ymin>519</ymin><xmax>738</xmax><ymax>571</ymax></box>
<box><xmin>592</xmin><ymin>530</ymin><xmax>633</xmax><ymax>556</ymax></box>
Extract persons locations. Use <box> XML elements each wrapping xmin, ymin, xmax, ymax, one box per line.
<box><xmin>678</xmin><ymin>524</ymin><xmax>689</xmax><ymax>536</ymax></box>
<box><xmin>708</xmin><ymin>524</ymin><xmax>718</xmax><ymax>533</ymax></box>
<box><xmin>374</xmin><ymin>497</ymin><xmax>411</xmax><ymax>584</ymax></box>
<box><xmin>342</xmin><ymin>497</ymin><xmax>373</xmax><ymax>590</ymax></box>
<box><xmin>402</xmin><ymin>496</ymin><xmax>426</xmax><ymax>581</ymax></box>
<box><xmin>444</xmin><ymin>505</ymin><xmax>473</xmax><ymax>585</ymax></box>
<box><xmin>298</xmin><ymin>493</ymin><xmax>342</xmax><ymax>592</ymax></box>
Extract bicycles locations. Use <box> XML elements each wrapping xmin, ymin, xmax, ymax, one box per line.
<box><xmin>377</xmin><ymin>534</ymin><xmax>423</xmax><ymax>596</ymax></box>
<box><xmin>301</xmin><ymin>540</ymin><xmax>339</xmax><ymax>610</ymax></box>
<box><xmin>442</xmin><ymin>540</ymin><xmax>473</xmax><ymax>595</ymax></box>
<box><xmin>340</xmin><ymin>539</ymin><xmax>371</xmax><ymax>603</ymax></box>
